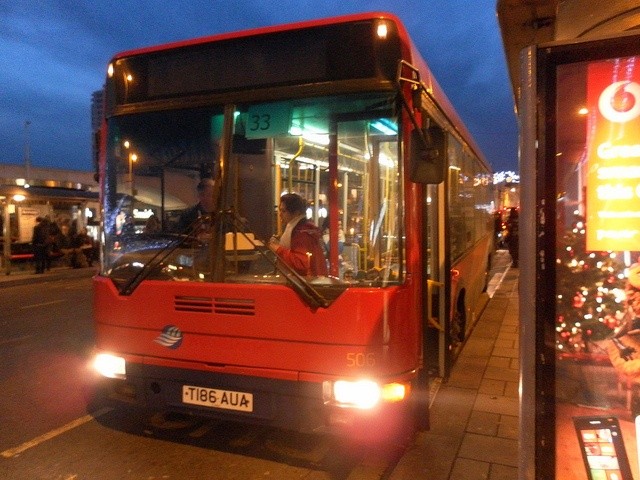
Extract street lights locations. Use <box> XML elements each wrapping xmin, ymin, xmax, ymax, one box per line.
<box><xmin>24</xmin><ymin>118</ymin><xmax>34</xmax><ymax>183</ymax></box>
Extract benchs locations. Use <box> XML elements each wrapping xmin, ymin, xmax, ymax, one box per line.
<box><xmin>8</xmin><ymin>253</ymin><xmax>63</xmax><ymax>260</ymax></box>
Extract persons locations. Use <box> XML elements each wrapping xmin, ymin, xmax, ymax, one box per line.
<box><xmin>266</xmin><ymin>193</ymin><xmax>328</xmax><ymax>276</ymax></box>
<box><xmin>494</xmin><ymin>212</ymin><xmax>502</xmax><ymax>250</ymax></box>
<box><xmin>32</xmin><ymin>215</ymin><xmax>95</xmax><ymax>274</ymax></box>
<box><xmin>320</xmin><ymin>216</ymin><xmax>346</xmax><ymax>268</ymax></box>
<box><xmin>504</xmin><ymin>207</ymin><xmax>518</xmax><ymax>268</ymax></box>
<box><xmin>180</xmin><ymin>178</ymin><xmax>219</xmax><ymax>249</ymax></box>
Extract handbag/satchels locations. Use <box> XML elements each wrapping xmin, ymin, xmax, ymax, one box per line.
<box><xmin>249</xmin><ymin>250</ymin><xmax>275</xmax><ymax>276</ymax></box>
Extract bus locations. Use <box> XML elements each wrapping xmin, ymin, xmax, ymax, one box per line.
<box><xmin>91</xmin><ymin>11</ymin><xmax>495</xmax><ymax>445</ymax></box>
